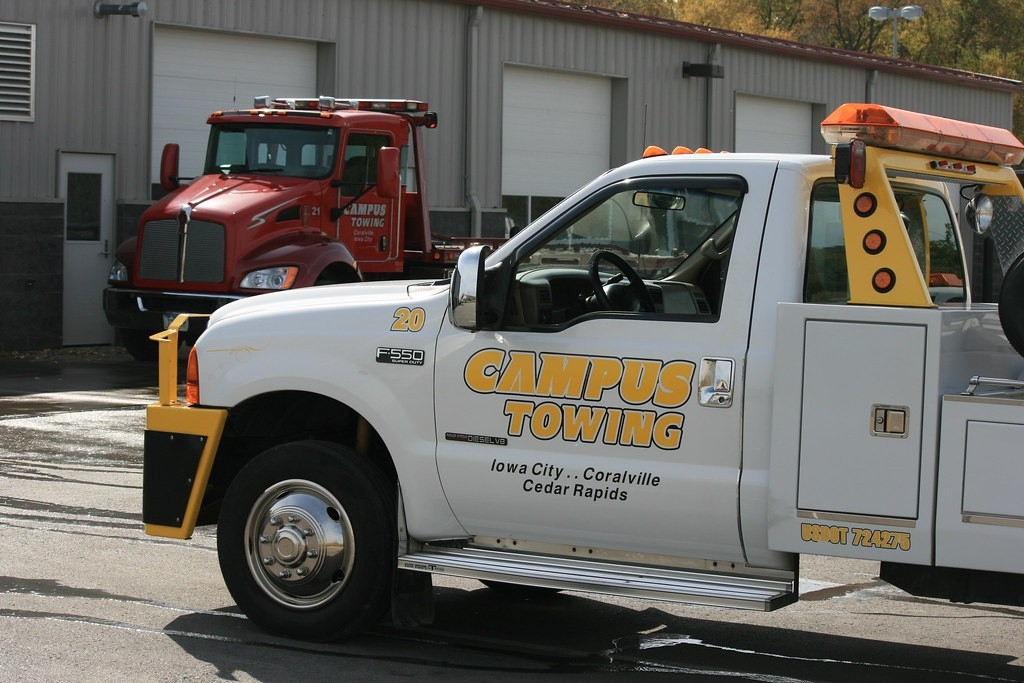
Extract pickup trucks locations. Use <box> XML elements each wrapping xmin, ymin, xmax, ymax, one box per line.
<box><xmin>134</xmin><ymin>100</ymin><xmax>1024</xmax><ymax>641</ymax></box>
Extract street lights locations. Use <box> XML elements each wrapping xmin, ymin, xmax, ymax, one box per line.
<box><xmin>867</xmin><ymin>4</ymin><xmax>924</xmax><ymax>60</ymax></box>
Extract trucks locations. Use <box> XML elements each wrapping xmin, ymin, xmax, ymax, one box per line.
<box><xmin>101</xmin><ymin>95</ymin><xmax>692</xmax><ymax>365</ymax></box>
